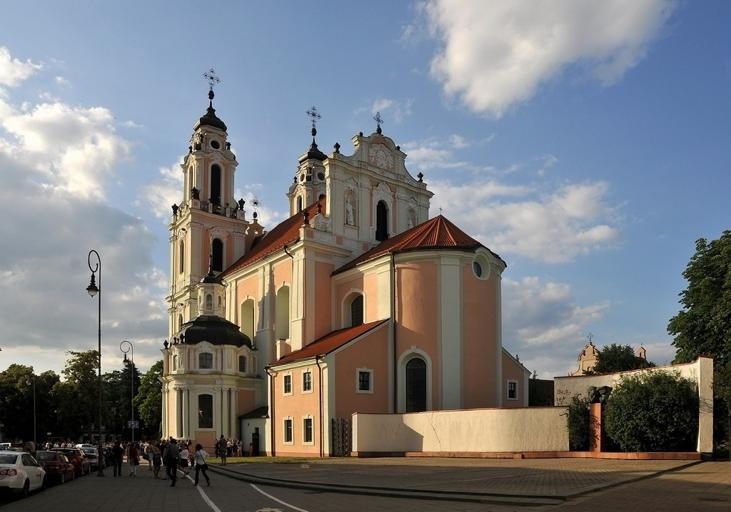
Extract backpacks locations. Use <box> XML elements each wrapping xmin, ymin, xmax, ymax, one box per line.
<box><xmin>165</xmin><ymin>444</ymin><xmax>180</xmax><ymax>462</ymax></box>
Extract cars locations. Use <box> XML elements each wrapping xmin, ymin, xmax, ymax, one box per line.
<box><xmin>1</xmin><ymin>441</ymin><xmax>113</xmax><ymax>501</ymax></box>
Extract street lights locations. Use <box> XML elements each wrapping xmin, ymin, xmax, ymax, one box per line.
<box><xmin>84</xmin><ymin>248</ymin><xmax>107</xmax><ymax>477</ymax></box>
<box><xmin>25</xmin><ymin>376</ymin><xmax>38</xmax><ymax>448</ymax></box>
<box><xmin>120</xmin><ymin>340</ymin><xmax>136</xmax><ymax>442</ymax></box>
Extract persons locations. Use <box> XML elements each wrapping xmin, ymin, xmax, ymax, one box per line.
<box><xmin>589</xmin><ymin>386</ymin><xmax>596</xmax><ymax>403</ymax></box>
<box><xmin>78</xmin><ymin>437</ymin><xmax>195</xmax><ymax>480</ymax></box>
<box><xmin>194</xmin><ymin>444</ymin><xmax>210</xmax><ymax>486</ymax></box>
<box><xmin>218</xmin><ymin>435</ymin><xmax>227</xmax><ymax>466</ymax></box>
<box><xmin>214</xmin><ymin>438</ymin><xmax>252</xmax><ymax>458</ymax></box>
<box><xmin>166</xmin><ymin>439</ymin><xmax>178</xmax><ymax>487</ymax></box>
<box><xmin>43</xmin><ymin>439</ymin><xmax>74</xmax><ymax>449</ymax></box>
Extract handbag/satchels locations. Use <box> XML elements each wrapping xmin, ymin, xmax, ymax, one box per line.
<box><xmin>133</xmin><ymin>458</ymin><xmax>139</xmax><ymax>465</ymax></box>
<box><xmin>202</xmin><ymin>464</ymin><xmax>209</xmax><ymax>470</ymax></box>
